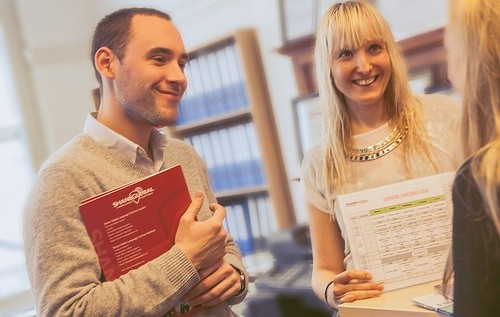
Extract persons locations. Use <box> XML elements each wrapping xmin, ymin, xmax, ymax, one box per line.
<box><xmin>23</xmin><ymin>6</ymin><xmax>249</xmax><ymax>316</ymax></box>
<box><xmin>298</xmin><ymin>0</ymin><xmax>469</xmax><ymax>317</ymax></box>
<box><xmin>438</xmin><ymin>0</ymin><xmax>499</xmax><ymax>316</ymax></box>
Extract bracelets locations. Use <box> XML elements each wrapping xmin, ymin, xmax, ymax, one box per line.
<box><xmin>324</xmin><ymin>280</ymin><xmax>338</xmax><ymax>311</ymax></box>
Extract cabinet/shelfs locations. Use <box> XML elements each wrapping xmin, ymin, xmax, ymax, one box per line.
<box><xmin>93</xmin><ymin>26</ymin><xmax>297</xmax><ymax>257</ymax></box>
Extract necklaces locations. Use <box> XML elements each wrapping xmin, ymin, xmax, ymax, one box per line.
<box><xmin>341</xmin><ymin>106</ymin><xmax>412</xmax><ymax>163</ymax></box>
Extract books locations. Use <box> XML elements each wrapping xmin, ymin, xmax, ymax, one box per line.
<box><xmin>410</xmin><ymin>287</ymin><xmax>455</xmax><ymax>315</ymax></box>
<box><xmin>77</xmin><ymin>162</ymin><xmax>215</xmax><ymax>316</ymax></box>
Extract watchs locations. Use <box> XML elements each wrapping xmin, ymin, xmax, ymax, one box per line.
<box><xmin>229</xmin><ymin>263</ymin><xmax>246</xmax><ymax>297</ymax></box>
